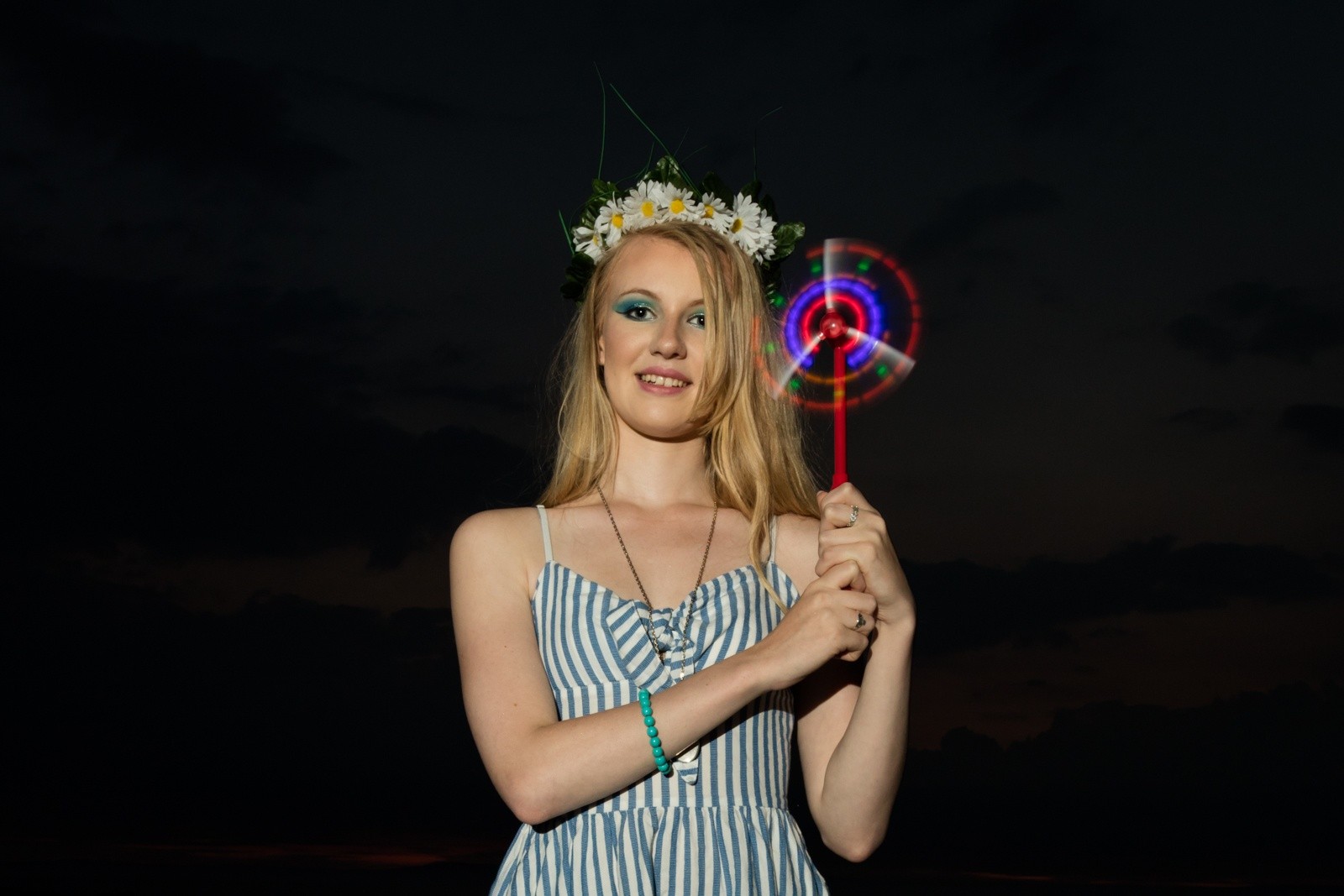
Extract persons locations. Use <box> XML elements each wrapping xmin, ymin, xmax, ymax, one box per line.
<box><xmin>447</xmin><ymin>222</ymin><xmax>917</xmax><ymax>896</ymax></box>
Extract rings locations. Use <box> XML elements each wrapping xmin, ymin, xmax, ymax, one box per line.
<box><xmin>850</xmin><ymin>610</ymin><xmax>867</xmax><ymax>630</ymax></box>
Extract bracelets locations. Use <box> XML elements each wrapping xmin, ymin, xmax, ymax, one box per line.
<box><xmin>638</xmin><ymin>689</ymin><xmax>672</xmax><ymax>775</ymax></box>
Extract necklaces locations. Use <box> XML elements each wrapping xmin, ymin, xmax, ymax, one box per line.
<box><xmin>593</xmin><ymin>478</ymin><xmax>717</xmax><ymax>680</ymax></box>
